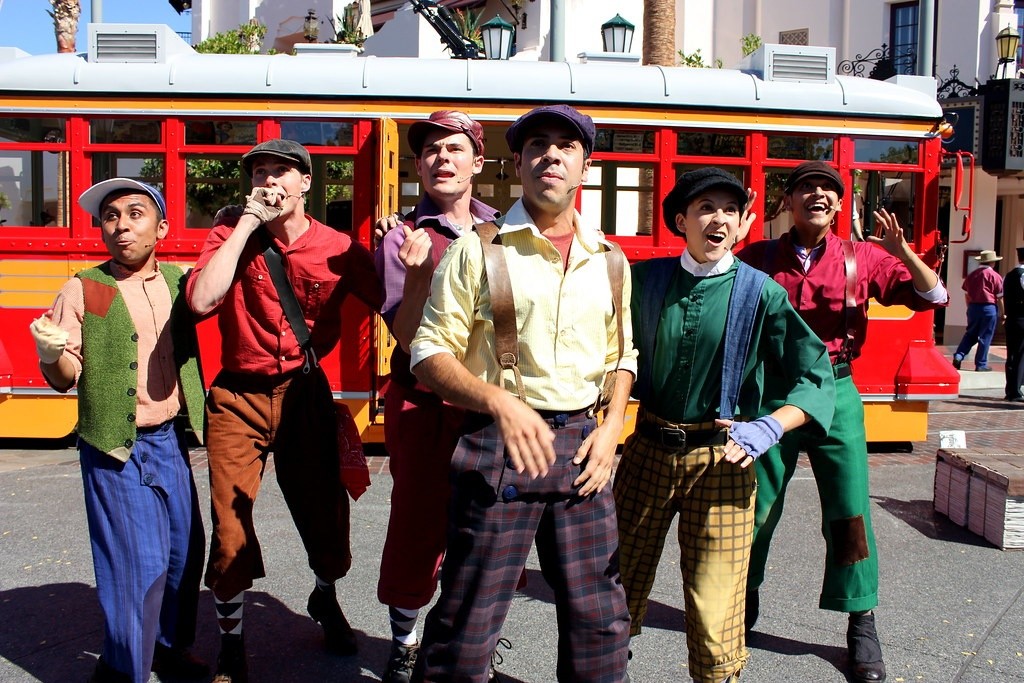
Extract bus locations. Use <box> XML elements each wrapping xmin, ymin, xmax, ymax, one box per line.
<box><xmin>0</xmin><ymin>22</ymin><xmax>975</xmax><ymax>445</ymax></box>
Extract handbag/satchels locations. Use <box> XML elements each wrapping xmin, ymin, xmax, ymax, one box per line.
<box><xmin>333</xmin><ymin>399</ymin><xmax>371</xmax><ymax>501</ymax></box>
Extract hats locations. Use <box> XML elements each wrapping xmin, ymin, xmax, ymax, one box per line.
<box><xmin>504</xmin><ymin>105</ymin><xmax>596</xmax><ymax>158</ymax></box>
<box><xmin>662</xmin><ymin>166</ymin><xmax>749</xmax><ymax>237</ymax></box>
<box><xmin>973</xmin><ymin>250</ymin><xmax>1004</xmax><ymax>263</ymax></box>
<box><xmin>784</xmin><ymin>160</ymin><xmax>844</xmax><ymax>198</ymax></box>
<box><xmin>240</xmin><ymin>139</ymin><xmax>313</xmax><ymax>176</ymax></box>
<box><xmin>77</xmin><ymin>177</ymin><xmax>167</xmax><ymax>220</ymax></box>
<box><xmin>407</xmin><ymin>110</ymin><xmax>486</xmax><ymax>156</ymax></box>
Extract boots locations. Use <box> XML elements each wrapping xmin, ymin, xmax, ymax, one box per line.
<box><xmin>382</xmin><ymin>636</ymin><xmax>419</xmax><ymax>683</ymax></box>
<box><xmin>216</xmin><ymin>630</ymin><xmax>249</xmax><ymax>683</ymax></box>
<box><xmin>845</xmin><ymin>612</ymin><xmax>886</xmax><ymax>683</ymax></box>
<box><xmin>306</xmin><ymin>585</ymin><xmax>358</xmax><ymax>656</ymax></box>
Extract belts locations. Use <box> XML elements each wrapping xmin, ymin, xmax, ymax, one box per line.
<box><xmin>635</xmin><ymin>419</ymin><xmax>728</xmax><ymax>447</ymax></box>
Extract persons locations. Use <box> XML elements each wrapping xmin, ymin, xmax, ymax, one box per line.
<box><xmin>730</xmin><ymin>160</ymin><xmax>950</xmax><ymax>683</ymax></box>
<box><xmin>613</xmin><ymin>168</ymin><xmax>837</xmax><ymax>681</ymax></box>
<box><xmin>376</xmin><ymin>110</ymin><xmax>501</xmax><ymax>682</ymax></box>
<box><xmin>411</xmin><ymin>104</ymin><xmax>640</xmax><ymax>683</ymax></box>
<box><xmin>184</xmin><ymin>140</ymin><xmax>408</xmax><ymax>683</ymax></box>
<box><xmin>1002</xmin><ymin>247</ymin><xmax>1024</xmax><ymax>402</ymax></box>
<box><xmin>952</xmin><ymin>249</ymin><xmax>1007</xmax><ymax>371</ymax></box>
<box><xmin>28</xmin><ymin>178</ymin><xmax>246</xmax><ymax>683</ymax></box>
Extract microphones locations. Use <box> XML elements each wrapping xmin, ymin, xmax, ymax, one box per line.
<box><xmin>571</xmin><ymin>182</ymin><xmax>583</xmax><ymax>189</ymax></box>
<box><xmin>287</xmin><ymin>194</ymin><xmax>304</xmax><ymax>199</ymax></box>
<box><xmin>144</xmin><ymin>241</ymin><xmax>158</xmax><ymax>249</ymax></box>
<box><xmin>457</xmin><ymin>173</ymin><xmax>474</xmax><ymax>184</ymax></box>
<box><xmin>724</xmin><ymin>239</ymin><xmax>736</xmax><ymax>252</ymax></box>
<box><xmin>829</xmin><ymin>205</ymin><xmax>836</xmax><ymax>212</ymax></box>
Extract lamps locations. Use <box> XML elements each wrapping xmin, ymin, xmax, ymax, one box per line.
<box><xmin>992</xmin><ymin>22</ymin><xmax>1021</xmax><ymax>79</ymax></box>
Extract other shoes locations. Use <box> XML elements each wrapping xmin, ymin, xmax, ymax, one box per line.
<box><xmin>953</xmin><ymin>358</ymin><xmax>961</xmax><ymax>370</ymax></box>
<box><xmin>92</xmin><ymin>652</ymin><xmax>139</xmax><ymax>683</ymax></box>
<box><xmin>153</xmin><ymin>644</ymin><xmax>207</xmax><ymax>672</ymax></box>
<box><xmin>1004</xmin><ymin>394</ymin><xmax>1024</xmax><ymax>402</ymax></box>
<box><xmin>976</xmin><ymin>366</ymin><xmax>992</xmax><ymax>371</ymax></box>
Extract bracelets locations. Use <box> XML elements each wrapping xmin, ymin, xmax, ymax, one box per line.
<box><xmin>1000</xmin><ymin>315</ymin><xmax>1007</xmax><ymax>321</ymax></box>
